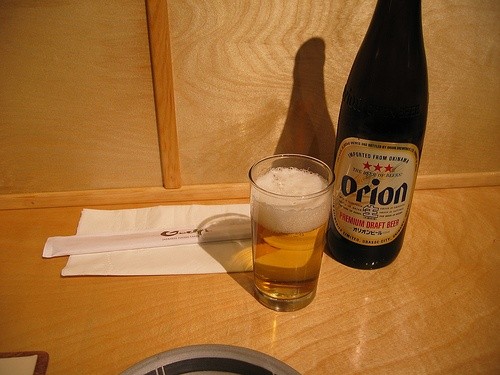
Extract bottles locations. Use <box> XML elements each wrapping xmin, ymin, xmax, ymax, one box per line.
<box><xmin>318</xmin><ymin>0</ymin><xmax>429</xmax><ymax>270</ymax></box>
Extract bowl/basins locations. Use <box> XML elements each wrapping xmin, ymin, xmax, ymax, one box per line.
<box><xmin>116</xmin><ymin>342</ymin><xmax>303</xmax><ymax>375</ymax></box>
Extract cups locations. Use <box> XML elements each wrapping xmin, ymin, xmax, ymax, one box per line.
<box><xmin>248</xmin><ymin>153</ymin><xmax>336</xmax><ymax>312</ymax></box>
